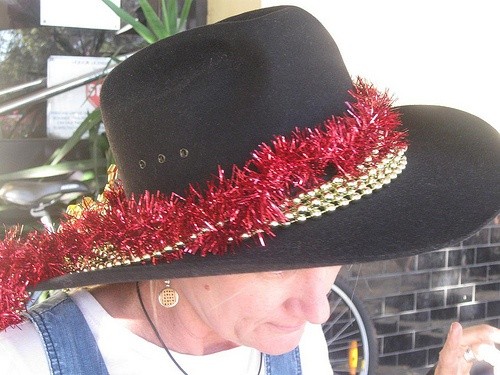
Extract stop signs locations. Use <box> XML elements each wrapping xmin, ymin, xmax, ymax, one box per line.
<box><xmin>85</xmin><ymin>69</ymin><xmax>105</xmax><ymax>108</ymax></box>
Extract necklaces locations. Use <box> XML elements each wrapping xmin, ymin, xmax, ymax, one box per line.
<box><xmin>134</xmin><ymin>280</ymin><xmax>263</xmax><ymax>374</ymax></box>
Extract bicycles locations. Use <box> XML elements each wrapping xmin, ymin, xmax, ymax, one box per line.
<box><xmin>0</xmin><ymin>177</ymin><xmax>376</xmax><ymax>374</ymax></box>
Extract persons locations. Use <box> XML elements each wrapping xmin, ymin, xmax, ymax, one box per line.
<box><xmin>1</xmin><ymin>4</ymin><xmax>500</xmax><ymax>374</ymax></box>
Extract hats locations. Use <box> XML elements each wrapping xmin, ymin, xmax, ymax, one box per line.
<box><xmin>24</xmin><ymin>4</ymin><xmax>500</xmax><ymax>292</ymax></box>
